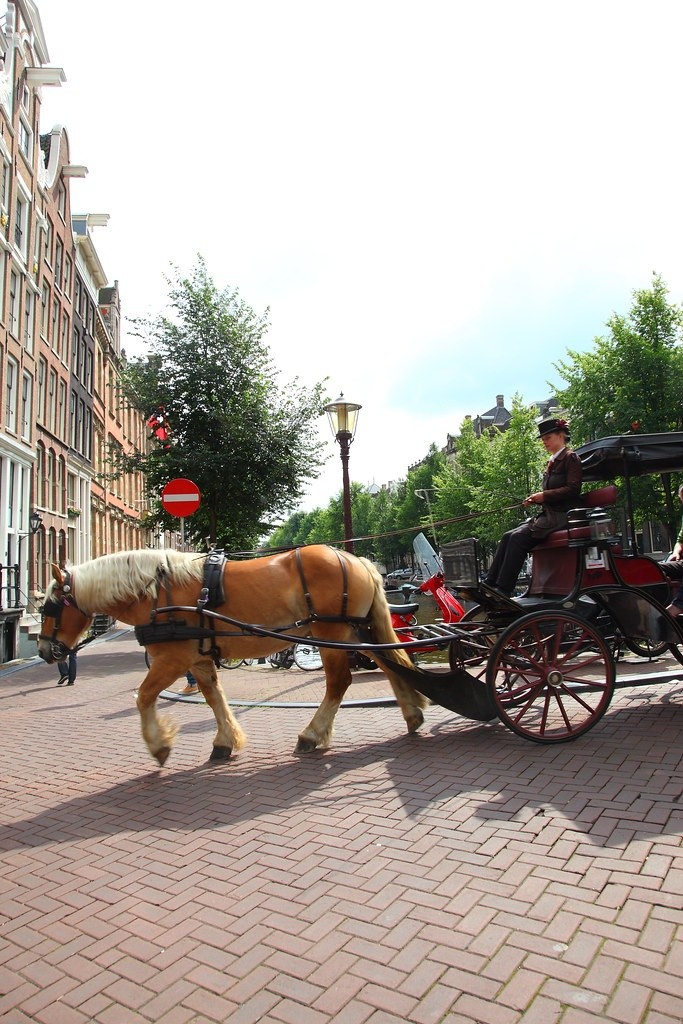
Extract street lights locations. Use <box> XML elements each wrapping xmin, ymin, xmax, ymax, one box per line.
<box><xmin>321</xmin><ymin>395</ymin><xmax>365</xmax><ymax>556</ymax></box>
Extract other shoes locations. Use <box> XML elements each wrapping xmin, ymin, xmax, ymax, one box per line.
<box><xmin>66</xmin><ymin>682</ymin><xmax>75</xmax><ymax>686</ymax></box>
<box><xmin>58</xmin><ymin>675</ymin><xmax>70</xmax><ymax>683</ymax></box>
<box><xmin>477</xmin><ymin>583</ymin><xmax>512</xmax><ymax>603</ymax></box>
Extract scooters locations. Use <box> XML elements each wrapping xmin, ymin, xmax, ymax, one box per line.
<box><xmin>352</xmin><ymin>532</ymin><xmax>488</xmax><ymax>670</ymax></box>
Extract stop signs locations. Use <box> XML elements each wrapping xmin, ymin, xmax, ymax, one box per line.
<box><xmin>161</xmin><ymin>479</ymin><xmax>201</xmax><ymax>518</ymax></box>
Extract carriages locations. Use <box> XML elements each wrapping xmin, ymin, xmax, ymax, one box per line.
<box><xmin>37</xmin><ymin>431</ymin><xmax>683</xmax><ymax>773</ymax></box>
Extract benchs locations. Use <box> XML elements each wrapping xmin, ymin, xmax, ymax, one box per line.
<box><xmin>528</xmin><ymin>484</ymin><xmax>619</xmax><ymax>553</ymax></box>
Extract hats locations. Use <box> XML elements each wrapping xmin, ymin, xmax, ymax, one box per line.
<box><xmin>536</xmin><ymin>419</ymin><xmax>569</xmax><ymax>439</ymax></box>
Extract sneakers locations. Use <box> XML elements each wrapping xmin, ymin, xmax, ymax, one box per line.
<box><xmin>176</xmin><ymin>684</ymin><xmax>201</xmax><ymax>695</ymax></box>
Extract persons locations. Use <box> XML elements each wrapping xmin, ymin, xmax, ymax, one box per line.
<box><xmin>658</xmin><ymin>485</ymin><xmax>683</xmax><ymax>579</ymax></box>
<box><xmin>178</xmin><ymin>671</ymin><xmax>203</xmax><ymax>695</ymax></box>
<box><xmin>478</xmin><ymin>414</ymin><xmax>584</xmax><ymax>598</ymax></box>
<box><xmin>57</xmin><ymin>643</ymin><xmax>77</xmax><ymax>685</ymax></box>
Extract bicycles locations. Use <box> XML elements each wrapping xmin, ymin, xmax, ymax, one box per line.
<box><xmin>217</xmin><ymin>656</ymin><xmax>254</xmax><ymax>669</ymax></box>
<box><xmin>266</xmin><ymin>633</ymin><xmax>325</xmax><ymax>670</ymax></box>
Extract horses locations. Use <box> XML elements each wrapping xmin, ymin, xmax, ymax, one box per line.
<box><xmin>37</xmin><ymin>543</ymin><xmax>431</xmax><ymax>768</ymax></box>
